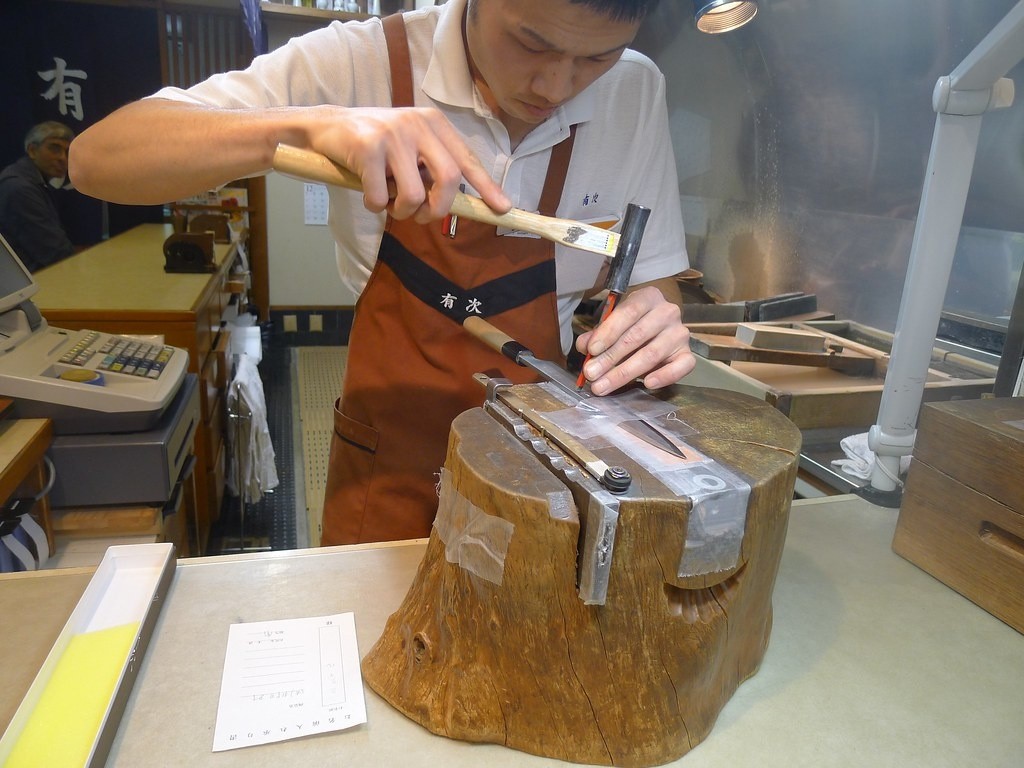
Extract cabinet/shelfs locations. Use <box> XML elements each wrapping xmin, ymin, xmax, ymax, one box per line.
<box><xmin>21</xmin><ymin>188</ymin><xmax>251</xmax><ymax>552</ymax></box>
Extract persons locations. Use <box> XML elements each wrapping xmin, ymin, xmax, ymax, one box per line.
<box><xmin>0</xmin><ymin>121</ymin><xmax>85</xmax><ymax>273</ymax></box>
<box><xmin>69</xmin><ymin>0</ymin><xmax>697</xmax><ymax>547</ymax></box>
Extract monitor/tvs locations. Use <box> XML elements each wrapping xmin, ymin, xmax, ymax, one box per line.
<box><xmin>0</xmin><ymin>234</ymin><xmax>37</xmax><ymax>313</ymax></box>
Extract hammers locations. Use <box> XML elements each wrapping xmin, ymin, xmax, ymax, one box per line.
<box><xmin>271</xmin><ymin>140</ymin><xmax>653</xmax><ymax>294</ymax></box>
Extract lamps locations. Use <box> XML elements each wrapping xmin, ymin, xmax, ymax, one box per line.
<box><xmin>694</xmin><ymin>0</ymin><xmax>758</xmax><ymax>34</ymax></box>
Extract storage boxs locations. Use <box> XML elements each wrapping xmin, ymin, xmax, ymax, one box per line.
<box><xmin>892</xmin><ymin>397</ymin><xmax>1024</xmax><ymax>640</ymax></box>
